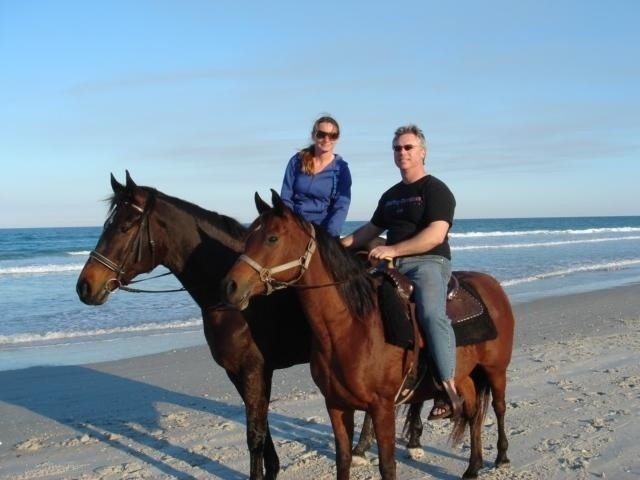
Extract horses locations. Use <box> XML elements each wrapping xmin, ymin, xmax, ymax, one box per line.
<box><xmin>75</xmin><ymin>169</ymin><xmax>426</xmax><ymax>480</ymax></box>
<box><xmin>216</xmin><ymin>187</ymin><xmax>516</xmax><ymax>480</ymax></box>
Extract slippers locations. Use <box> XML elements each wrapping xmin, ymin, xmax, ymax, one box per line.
<box><xmin>427</xmin><ymin>395</ymin><xmax>464</xmax><ymax>420</ymax></box>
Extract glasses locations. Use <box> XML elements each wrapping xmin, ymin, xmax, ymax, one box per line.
<box><xmin>315</xmin><ymin>130</ymin><xmax>338</xmax><ymax>139</ymax></box>
<box><xmin>394</xmin><ymin>144</ymin><xmax>416</xmax><ymax>151</ymax></box>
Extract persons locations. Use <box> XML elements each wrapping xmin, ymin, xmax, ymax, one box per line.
<box><xmin>339</xmin><ymin>124</ymin><xmax>467</xmax><ymax>417</ymax></box>
<box><xmin>280</xmin><ymin>115</ymin><xmax>352</xmax><ymax>242</ymax></box>
